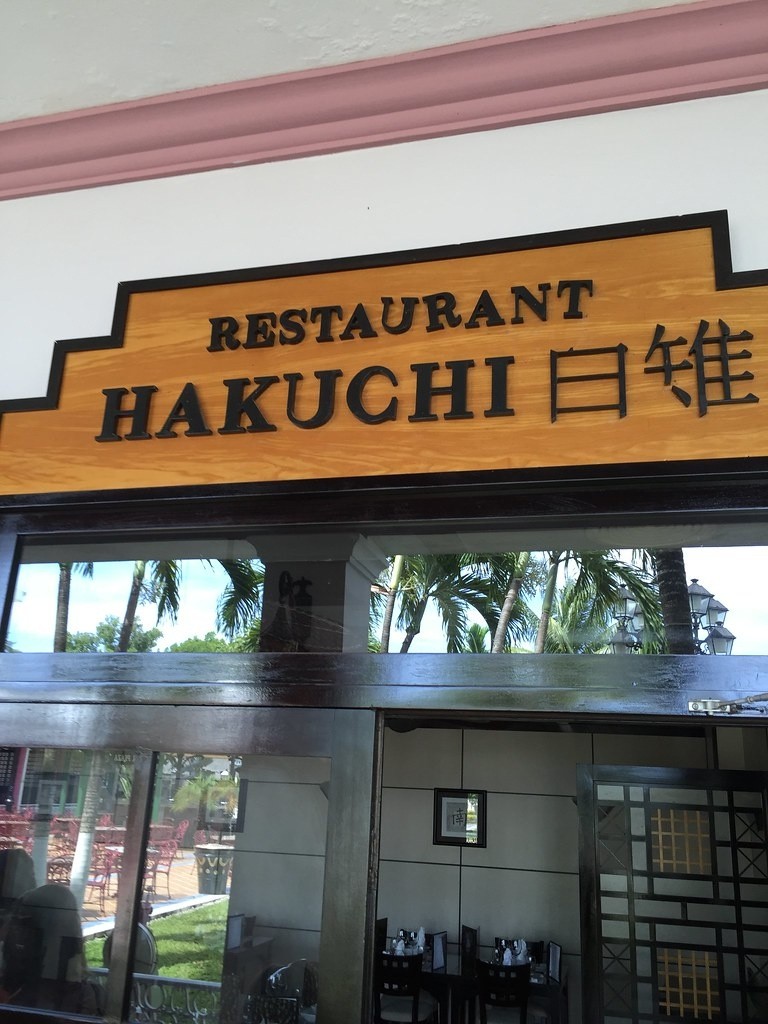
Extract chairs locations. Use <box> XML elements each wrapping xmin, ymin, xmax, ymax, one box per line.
<box><xmin>45</xmin><ymin>839</ymin><xmax>177</xmax><ymax>914</ymax></box>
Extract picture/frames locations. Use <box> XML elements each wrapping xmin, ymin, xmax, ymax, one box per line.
<box><xmin>548</xmin><ymin>941</ymin><xmax>562</xmax><ymax>982</ymax></box>
<box><xmin>432</xmin><ymin>931</ymin><xmax>446</xmax><ymax>971</ymax></box>
<box><xmin>433</xmin><ymin>787</ymin><xmax>487</xmax><ymax>848</ymax></box>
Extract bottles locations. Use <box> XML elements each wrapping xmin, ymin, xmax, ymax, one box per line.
<box><xmin>389</xmin><ymin>929</ymin><xmax>425</xmax><ymax>958</ymax></box>
<box><xmin>492</xmin><ymin>936</ymin><xmax>527</xmax><ymax>970</ymax></box>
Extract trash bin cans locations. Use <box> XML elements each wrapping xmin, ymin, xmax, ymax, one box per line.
<box><xmin>193</xmin><ymin>844</ymin><xmax>236</xmax><ymax>895</ymax></box>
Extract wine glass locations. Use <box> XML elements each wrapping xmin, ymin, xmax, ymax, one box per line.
<box><xmin>527</xmin><ymin>955</ymin><xmax>536</xmax><ymax>978</ymax></box>
